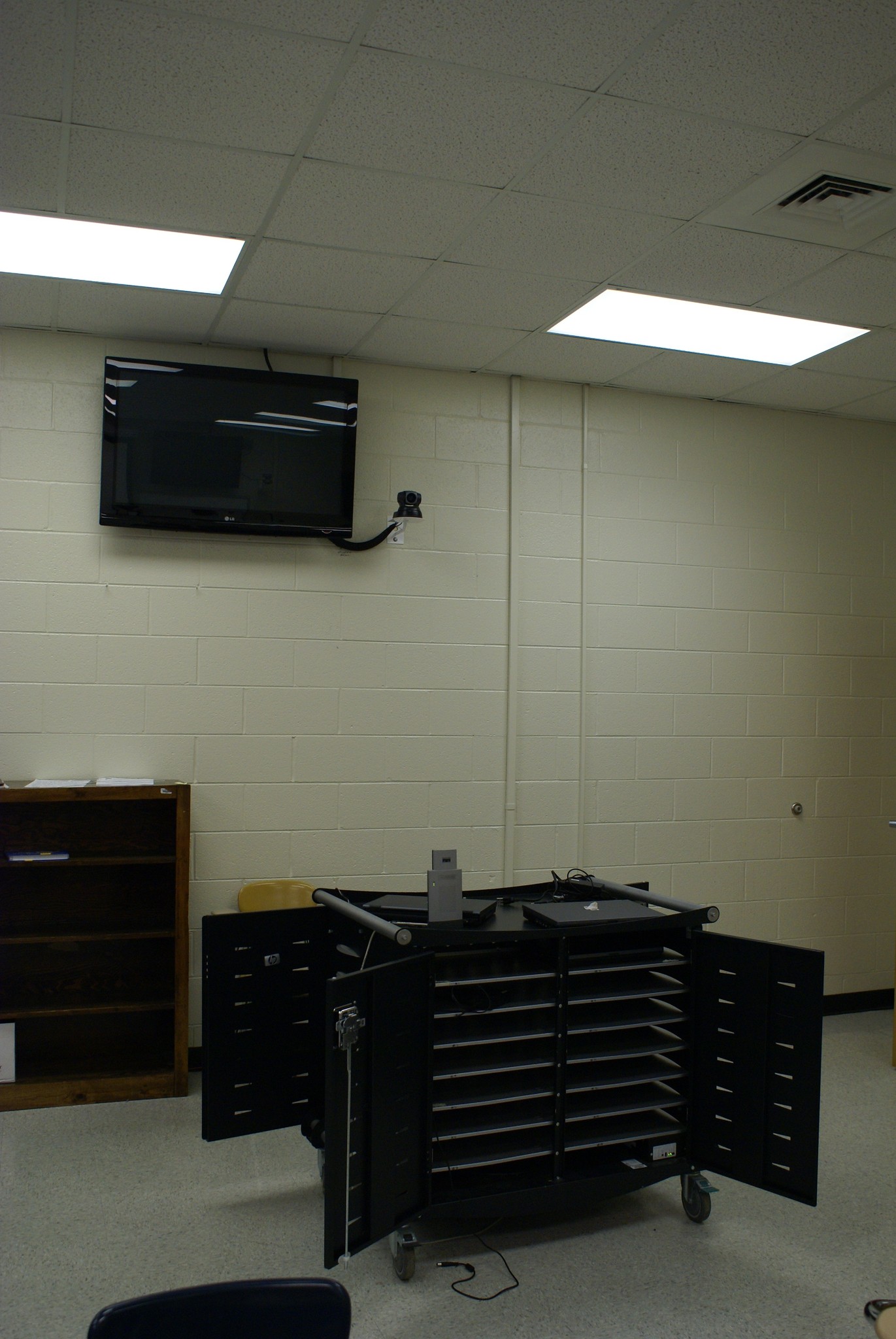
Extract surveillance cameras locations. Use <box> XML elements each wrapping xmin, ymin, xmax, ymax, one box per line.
<box><xmin>392</xmin><ymin>491</ymin><xmax>423</xmax><ymax>519</ymax></box>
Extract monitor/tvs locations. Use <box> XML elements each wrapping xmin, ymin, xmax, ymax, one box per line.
<box><xmin>100</xmin><ymin>356</ymin><xmax>358</xmax><ymax>540</ymax></box>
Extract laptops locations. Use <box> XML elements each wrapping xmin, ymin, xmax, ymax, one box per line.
<box><xmin>522</xmin><ymin>899</ymin><xmax>668</xmax><ymax>930</ymax></box>
<box><xmin>361</xmin><ymin>894</ymin><xmax>498</xmax><ymax>927</ymax></box>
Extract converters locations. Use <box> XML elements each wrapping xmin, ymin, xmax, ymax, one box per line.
<box><xmin>566</xmin><ymin>878</ymin><xmax>605</xmax><ymax>895</ymax></box>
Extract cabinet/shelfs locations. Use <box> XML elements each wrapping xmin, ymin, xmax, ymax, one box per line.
<box><xmin>199</xmin><ymin>874</ymin><xmax>825</xmax><ymax>1281</ymax></box>
<box><xmin>0</xmin><ymin>778</ymin><xmax>191</xmax><ymax>1113</ymax></box>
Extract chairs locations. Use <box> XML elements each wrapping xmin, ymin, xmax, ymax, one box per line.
<box><xmin>86</xmin><ymin>1276</ymin><xmax>353</xmax><ymax>1339</ymax></box>
<box><xmin>211</xmin><ymin>881</ymin><xmax>317</xmax><ymax>915</ymax></box>
